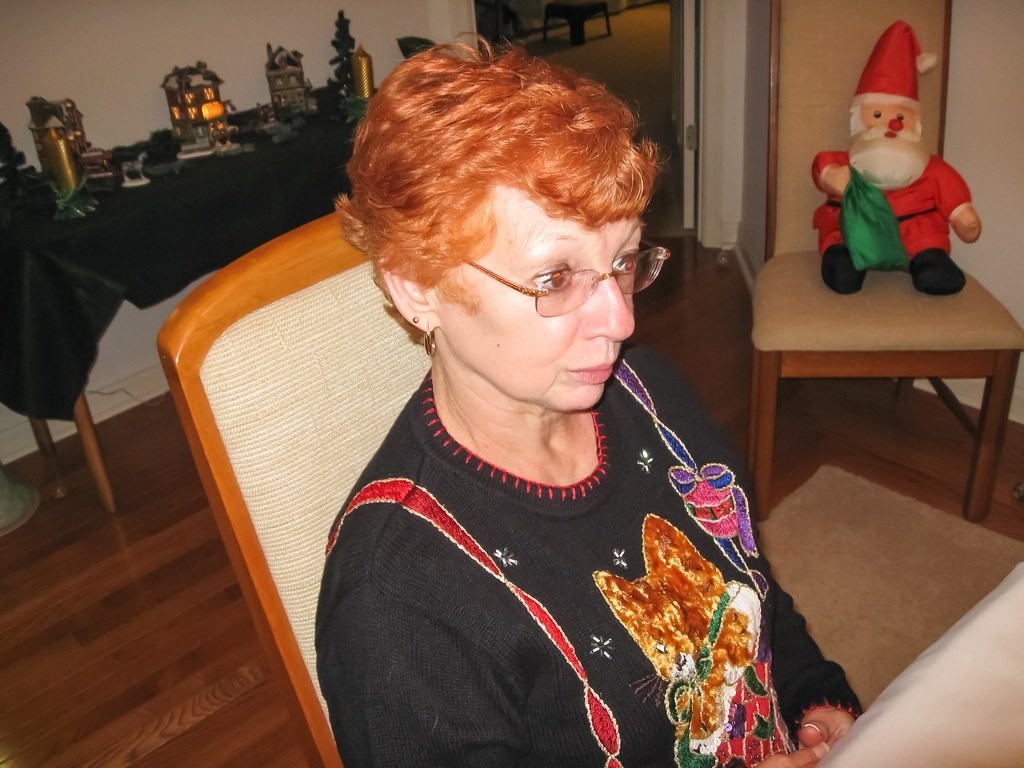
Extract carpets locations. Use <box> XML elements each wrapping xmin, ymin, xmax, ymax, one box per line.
<box><xmin>760</xmin><ymin>463</ymin><xmax>1024</xmax><ymax>704</ymax></box>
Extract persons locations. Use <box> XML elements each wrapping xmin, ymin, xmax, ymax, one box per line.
<box><xmin>811</xmin><ymin>22</ymin><xmax>981</xmax><ymax>295</ymax></box>
<box><xmin>313</xmin><ymin>32</ymin><xmax>863</xmax><ymax>768</ymax></box>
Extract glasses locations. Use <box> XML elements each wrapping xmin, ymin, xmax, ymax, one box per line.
<box><xmin>464</xmin><ymin>238</ymin><xmax>670</xmax><ymax>318</ymax></box>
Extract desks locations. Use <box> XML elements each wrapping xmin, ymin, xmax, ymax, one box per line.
<box><xmin>1</xmin><ymin>86</ymin><xmax>378</xmax><ymax>517</ymax></box>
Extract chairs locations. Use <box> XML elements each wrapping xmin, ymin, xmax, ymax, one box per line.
<box><xmin>750</xmin><ymin>0</ymin><xmax>1024</xmax><ymax>526</ymax></box>
<box><xmin>154</xmin><ymin>208</ymin><xmax>432</xmax><ymax>768</ymax></box>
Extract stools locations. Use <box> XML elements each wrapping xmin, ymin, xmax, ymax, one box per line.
<box><xmin>543</xmin><ymin>0</ymin><xmax>612</xmax><ymax>46</ymax></box>
